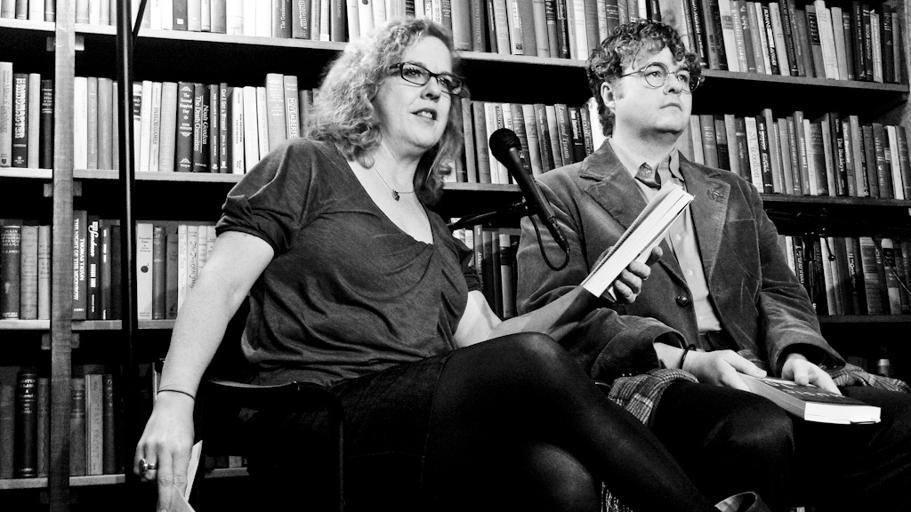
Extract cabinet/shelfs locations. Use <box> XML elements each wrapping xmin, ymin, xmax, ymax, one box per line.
<box><xmin>1</xmin><ymin>0</ymin><xmax>911</xmax><ymax>508</ymax></box>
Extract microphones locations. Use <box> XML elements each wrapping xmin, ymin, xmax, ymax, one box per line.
<box><xmin>488</xmin><ymin>127</ymin><xmax>570</xmax><ymax>251</ymax></box>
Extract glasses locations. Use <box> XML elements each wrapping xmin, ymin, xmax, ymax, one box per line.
<box><xmin>619</xmin><ymin>61</ymin><xmax>701</xmax><ymax>93</ymax></box>
<box><xmin>389</xmin><ymin>62</ymin><xmax>465</xmax><ymax>96</ymax></box>
